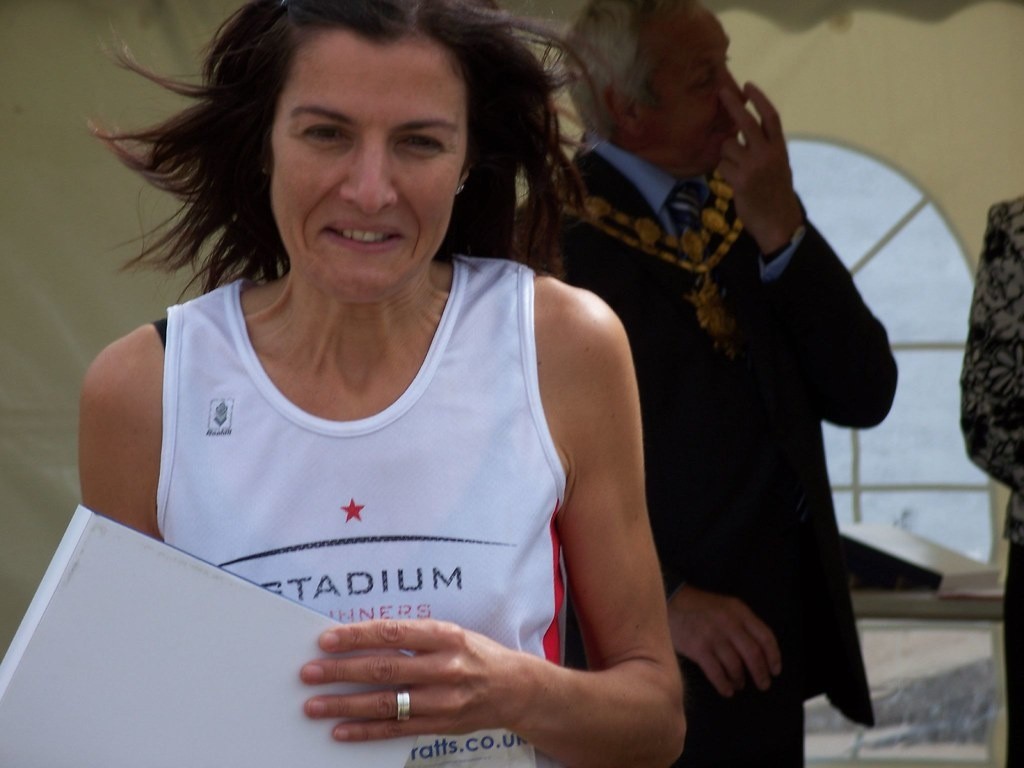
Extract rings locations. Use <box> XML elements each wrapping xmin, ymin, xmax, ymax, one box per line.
<box><xmin>393</xmin><ymin>688</ymin><xmax>411</xmax><ymax>725</ymax></box>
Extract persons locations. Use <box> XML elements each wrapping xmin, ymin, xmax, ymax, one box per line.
<box><xmin>79</xmin><ymin>0</ymin><xmax>696</xmax><ymax>768</ymax></box>
<box><xmin>509</xmin><ymin>0</ymin><xmax>898</xmax><ymax>768</ymax></box>
<box><xmin>958</xmin><ymin>199</ymin><xmax>1022</xmax><ymax>762</ymax></box>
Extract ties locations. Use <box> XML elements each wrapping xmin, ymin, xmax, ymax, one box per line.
<box><xmin>670</xmin><ymin>185</ymin><xmax>704</xmax><ymax>240</ymax></box>
